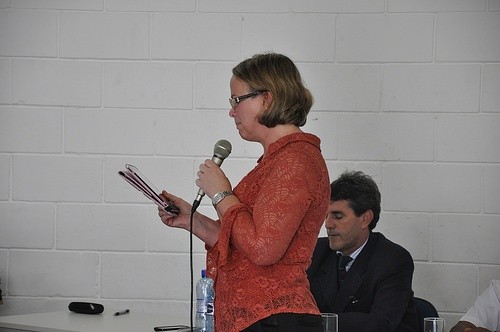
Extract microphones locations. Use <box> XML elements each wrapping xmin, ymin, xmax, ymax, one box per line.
<box><xmin>192</xmin><ymin>139</ymin><xmax>232</xmax><ymax>214</ymax></box>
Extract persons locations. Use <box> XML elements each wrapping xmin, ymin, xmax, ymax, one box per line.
<box><xmin>159</xmin><ymin>52</ymin><xmax>331</xmax><ymax>332</ymax></box>
<box><xmin>306</xmin><ymin>169</ymin><xmax>414</xmax><ymax>332</ymax></box>
<box><xmin>450</xmin><ymin>279</ymin><xmax>500</xmax><ymax>332</ymax></box>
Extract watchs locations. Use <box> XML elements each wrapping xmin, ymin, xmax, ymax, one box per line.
<box><xmin>212</xmin><ymin>190</ymin><xmax>234</xmax><ymax>209</ymax></box>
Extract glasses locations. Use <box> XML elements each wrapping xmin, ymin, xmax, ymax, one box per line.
<box><xmin>229</xmin><ymin>89</ymin><xmax>269</xmax><ymax>108</ymax></box>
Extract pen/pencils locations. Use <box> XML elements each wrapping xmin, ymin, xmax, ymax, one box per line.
<box><xmin>114</xmin><ymin>309</ymin><xmax>130</xmax><ymax>316</ymax></box>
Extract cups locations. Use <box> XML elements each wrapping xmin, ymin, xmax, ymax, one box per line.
<box><xmin>321</xmin><ymin>313</ymin><xmax>339</xmax><ymax>332</ymax></box>
<box><xmin>424</xmin><ymin>317</ymin><xmax>445</xmax><ymax>332</ymax></box>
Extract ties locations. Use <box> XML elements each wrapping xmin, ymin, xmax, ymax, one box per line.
<box><xmin>336</xmin><ymin>255</ymin><xmax>353</xmax><ymax>292</ymax></box>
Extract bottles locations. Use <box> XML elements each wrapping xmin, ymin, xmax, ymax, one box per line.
<box><xmin>196</xmin><ymin>270</ymin><xmax>215</xmax><ymax>332</ymax></box>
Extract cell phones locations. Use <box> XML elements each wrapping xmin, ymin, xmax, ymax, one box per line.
<box><xmin>154</xmin><ymin>325</ymin><xmax>189</xmax><ymax>332</ymax></box>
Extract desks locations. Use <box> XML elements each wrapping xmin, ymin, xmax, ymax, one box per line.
<box><xmin>0</xmin><ymin>310</ymin><xmax>197</xmax><ymax>332</ymax></box>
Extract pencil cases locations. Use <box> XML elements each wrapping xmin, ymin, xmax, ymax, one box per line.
<box><xmin>67</xmin><ymin>302</ymin><xmax>104</xmax><ymax>313</ymax></box>
